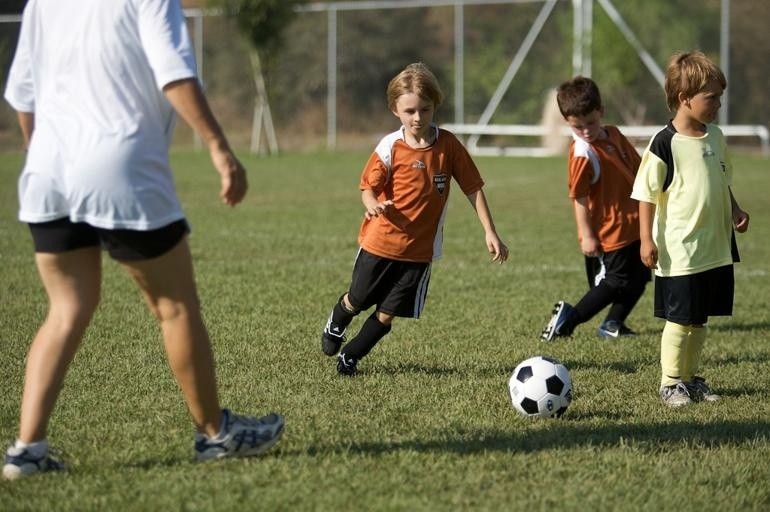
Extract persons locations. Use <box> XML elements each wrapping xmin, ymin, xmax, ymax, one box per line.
<box><xmin>539</xmin><ymin>75</ymin><xmax>653</xmax><ymax>347</ymax></box>
<box><xmin>1</xmin><ymin>0</ymin><xmax>283</xmax><ymax>485</ymax></box>
<box><xmin>630</xmin><ymin>48</ymin><xmax>750</xmax><ymax>409</ymax></box>
<box><xmin>322</xmin><ymin>62</ymin><xmax>509</xmax><ymax>375</ymax></box>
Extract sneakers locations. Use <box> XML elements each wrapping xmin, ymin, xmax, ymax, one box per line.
<box><xmin>193</xmin><ymin>407</ymin><xmax>285</xmax><ymax>462</ymax></box>
<box><xmin>659</xmin><ymin>382</ymin><xmax>692</xmax><ymax>407</ymax></box>
<box><xmin>1</xmin><ymin>447</ymin><xmax>62</xmax><ymax>481</ymax></box>
<box><xmin>539</xmin><ymin>300</ymin><xmax>576</xmax><ymax>342</ymax></box>
<box><xmin>597</xmin><ymin>320</ymin><xmax>636</xmax><ymax>338</ymax></box>
<box><xmin>321</xmin><ymin>306</ymin><xmax>348</xmax><ymax>355</ymax></box>
<box><xmin>337</xmin><ymin>344</ymin><xmax>358</xmax><ymax>374</ymax></box>
<box><xmin>687</xmin><ymin>378</ymin><xmax>722</xmax><ymax>405</ymax></box>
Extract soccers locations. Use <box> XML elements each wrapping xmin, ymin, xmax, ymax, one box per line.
<box><xmin>509</xmin><ymin>356</ymin><xmax>572</xmax><ymax>418</ymax></box>
